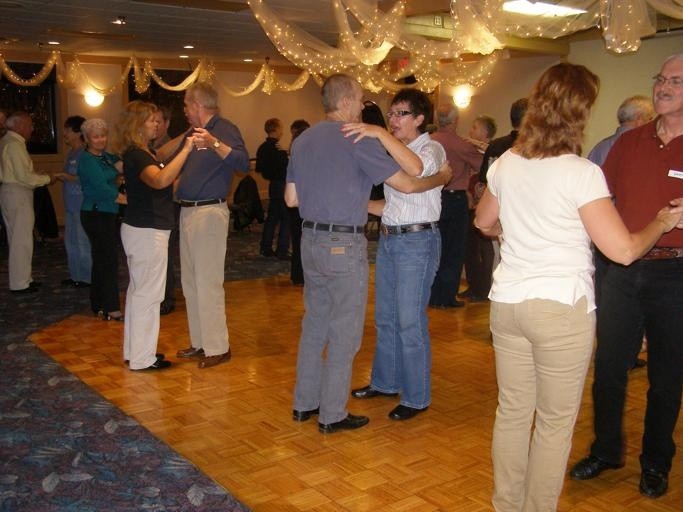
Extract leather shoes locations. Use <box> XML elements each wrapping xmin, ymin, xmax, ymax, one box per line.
<box><xmin>471</xmin><ymin>295</ymin><xmax>486</xmax><ymax>300</ymax></box>
<box><xmin>146</xmin><ymin>360</ymin><xmax>171</xmax><ymax>369</ymax></box>
<box><xmin>31</xmin><ymin>279</ymin><xmax>42</xmax><ymax>284</ymax></box>
<box><xmin>319</xmin><ymin>412</ymin><xmax>369</xmax><ymax>432</ymax></box>
<box><xmin>11</xmin><ymin>285</ymin><xmax>37</xmax><ymax>292</ymax></box>
<box><xmin>156</xmin><ymin>353</ymin><xmax>166</xmax><ymax>359</ymax></box>
<box><xmin>639</xmin><ymin>468</ymin><xmax>670</xmax><ymax>498</ymax></box>
<box><xmin>177</xmin><ymin>347</ymin><xmax>203</xmax><ymax>359</ymax></box>
<box><xmin>260</xmin><ymin>247</ymin><xmax>275</xmax><ymax>257</ymax></box>
<box><xmin>353</xmin><ymin>385</ymin><xmax>400</xmax><ymax>398</ymax></box>
<box><xmin>389</xmin><ymin>405</ymin><xmax>429</xmax><ymax>421</ymax></box>
<box><xmin>69</xmin><ymin>281</ymin><xmax>88</xmax><ymax>286</ymax></box>
<box><xmin>292</xmin><ymin>408</ymin><xmax>321</xmax><ymax>421</ymax></box>
<box><xmin>570</xmin><ymin>455</ymin><xmax>624</xmax><ymax>480</ymax></box>
<box><xmin>428</xmin><ymin>301</ymin><xmax>466</xmax><ymax>309</ymax></box>
<box><xmin>458</xmin><ymin>288</ymin><xmax>474</xmax><ymax>298</ymax></box>
<box><xmin>160</xmin><ymin>301</ymin><xmax>174</xmax><ymax>315</ymax></box>
<box><xmin>199</xmin><ymin>346</ymin><xmax>232</xmax><ymax>369</ymax></box>
<box><xmin>634</xmin><ymin>358</ymin><xmax>646</xmax><ymax>368</ymax></box>
<box><xmin>62</xmin><ymin>278</ymin><xmax>72</xmax><ymax>284</ymax></box>
<box><xmin>276</xmin><ymin>246</ymin><xmax>292</xmax><ymax>260</ymax></box>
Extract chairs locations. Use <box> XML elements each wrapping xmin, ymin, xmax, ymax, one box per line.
<box><xmin>227</xmin><ymin>174</ymin><xmax>259</xmax><ymax>233</ymax></box>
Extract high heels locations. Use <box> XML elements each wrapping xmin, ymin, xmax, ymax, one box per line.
<box><xmin>92</xmin><ymin>308</ymin><xmax>104</xmax><ymax>316</ymax></box>
<box><xmin>103</xmin><ymin>311</ymin><xmax>125</xmax><ymax>321</ymax></box>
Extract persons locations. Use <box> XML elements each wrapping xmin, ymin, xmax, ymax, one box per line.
<box><xmin>54</xmin><ymin>115</ymin><xmax>93</xmax><ymax>287</ymax></box>
<box><xmin>254</xmin><ymin>118</ymin><xmax>292</xmax><ymax>261</ymax></box>
<box><xmin>275</xmin><ymin>119</ymin><xmax>310</xmax><ymax>285</ymax></box>
<box><xmin>569</xmin><ymin>52</ymin><xmax>683</xmax><ymax>500</ymax></box>
<box><xmin>475</xmin><ymin>62</ymin><xmax>680</xmax><ymax>512</ymax></box>
<box><xmin>76</xmin><ymin>118</ymin><xmax>128</xmax><ymax>322</ymax></box>
<box><xmin>176</xmin><ymin>81</ymin><xmax>252</xmax><ymax>369</ymax></box>
<box><xmin>115</xmin><ymin>100</ymin><xmax>196</xmax><ymax>371</ymax></box>
<box><xmin>430</xmin><ymin>102</ymin><xmax>484</xmax><ymax>309</ymax></box>
<box><xmin>457</xmin><ymin>114</ymin><xmax>497</xmax><ymax>303</ymax></box>
<box><xmin>480</xmin><ymin>97</ymin><xmax>530</xmax><ymax>289</ymax></box>
<box><xmin>340</xmin><ymin>87</ymin><xmax>448</xmax><ymax>421</ymax></box>
<box><xmin>586</xmin><ymin>94</ymin><xmax>655</xmax><ymax>371</ymax></box>
<box><xmin>283</xmin><ymin>73</ymin><xmax>453</xmax><ymax>433</ymax></box>
<box><xmin>150</xmin><ymin>105</ymin><xmax>180</xmax><ymax>316</ymax></box>
<box><xmin>0</xmin><ymin>110</ymin><xmax>58</xmax><ymax>294</ymax></box>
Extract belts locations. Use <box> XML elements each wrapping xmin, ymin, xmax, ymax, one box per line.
<box><xmin>442</xmin><ymin>189</ymin><xmax>465</xmax><ymax>194</ymax></box>
<box><xmin>180</xmin><ymin>199</ymin><xmax>225</xmax><ymax>207</ymax></box>
<box><xmin>642</xmin><ymin>247</ymin><xmax>681</xmax><ymax>259</ymax></box>
<box><xmin>300</xmin><ymin>221</ymin><xmax>366</xmax><ymax>234</ymax></box>
<box><xmin>378</xmin><ymin>222</ymin><xmax>440</xmax><ymax>233</ymax></box>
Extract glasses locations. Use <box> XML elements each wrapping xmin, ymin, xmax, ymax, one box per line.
<box><xmin>652</xmin><ymin>74</ymin><xmax>682</xmax><ymax>85</ymax></box>
<box><xmin>385</xmin><ymin>110</ymin><xmax>412</xmax><ymax>117</ymax></box>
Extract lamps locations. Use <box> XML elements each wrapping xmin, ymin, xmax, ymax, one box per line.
<box><xmin>453</xmin><ymin>85</ymin><xmax>472</xmax><ymax>108</ymax></box>
<box><xmin>85</xmin><ymin>89</ymin><xmax>105</xmax><ymax>108</ymax></box>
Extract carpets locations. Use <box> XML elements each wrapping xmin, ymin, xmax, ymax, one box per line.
<box><xmin>1</xmin><ymin>208</ymin><xmax>384</xmax><ymax>336</ymax></box>
<box><xmin>1</xmin><ymin>336</ymin><xmax>250</xmax><ymax>506</ymax></box>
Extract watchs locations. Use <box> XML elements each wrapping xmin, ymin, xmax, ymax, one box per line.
<box><xmin>212</xmin><ymin>139</ymin><xmax>221</xmax><ymax>151</ymax></box>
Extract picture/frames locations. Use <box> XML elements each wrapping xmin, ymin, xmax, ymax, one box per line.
<box><xmin>127</xmin><ymin>67</ymin><xmax>197</xmax><ymax>139</ymax></box>
<box><xmin>0</xmin><ymin>61</ymin><xmax>58</xmax><ymax>155</ymax></box>
